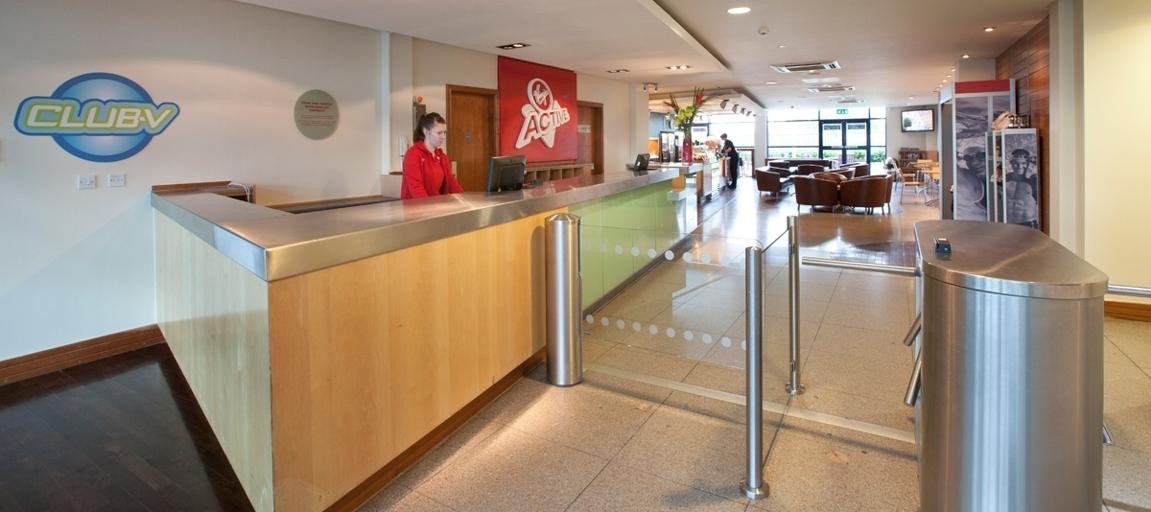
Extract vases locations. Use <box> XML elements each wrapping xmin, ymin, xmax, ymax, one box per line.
<box><xmin>682</xmin><ymin>127</ymin><xmax>694</xmax><ymax>166</ymax></box>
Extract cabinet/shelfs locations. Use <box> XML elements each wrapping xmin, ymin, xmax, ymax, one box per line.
<box><xmin>985</xmin><ymin>128</ymin><xmax>1043</xmax><ymax>233</ymax></box>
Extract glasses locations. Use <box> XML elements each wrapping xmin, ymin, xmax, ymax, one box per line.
<box><xmin>963</xmin><ymin>152</ymin><xmax>986</xmax><ymax>162</ymax></box>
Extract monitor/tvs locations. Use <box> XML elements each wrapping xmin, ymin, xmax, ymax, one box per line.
<box><xmin>901</xmin><ymin>109</ymin><xmax>934</xmax><ymax>132</ymax></box>
<box><xmin>634</xmin><ymin>153</ymin><xmax>650</xmax><ymax>170</ymax></box>
<box><xmin>486</xmin><ymin>154</ymin><xmax>526</xmax><ymax>192</ymax></box>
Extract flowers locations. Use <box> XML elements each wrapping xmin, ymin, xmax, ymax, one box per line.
<box><xmin>662</xmin><ymin>87</ymin><xmax>718</xmax><ymax>134</ymax></box>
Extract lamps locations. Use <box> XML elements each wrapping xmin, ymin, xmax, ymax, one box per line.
<box><xmin>720</xmin><ymin>99</ymin><xmax>758</xmax><ymax>118</ymax></box>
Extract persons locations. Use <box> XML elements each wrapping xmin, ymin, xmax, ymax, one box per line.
<box><xmin>956</xmin><ymin>138</ymin><xmax>987</xmax><ymax>222</ymax></box>
<box><xmin>1006</xmin><ymin>149</ymin><xmax>1038</xmax><ymax>230</ymax></box>
<box><xmin>400</xmin><ymin>113</ymin><xmax>464</xmax><ymax>199</ymax></box>
<box><xmin>720</xmin><ymin>133</ymin><xmax>738</xmax><ymax>190</ymax></box>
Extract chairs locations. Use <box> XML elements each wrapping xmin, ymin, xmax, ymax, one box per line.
<box><xmin>758</xmin><ymin>157</ymin><xmax>940</xmax><ymax>218</ymax></box>
